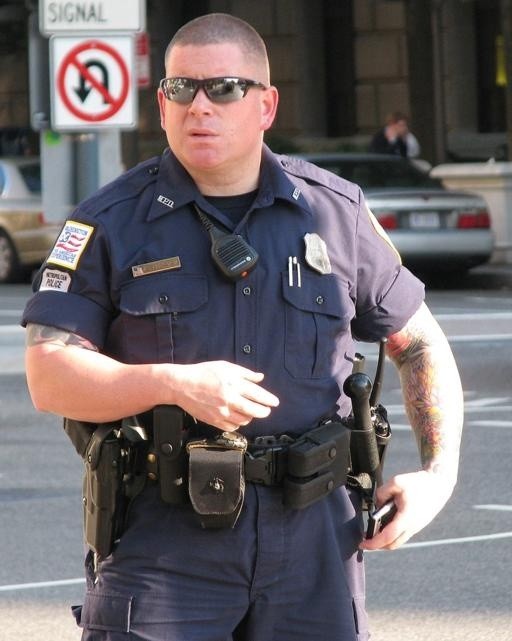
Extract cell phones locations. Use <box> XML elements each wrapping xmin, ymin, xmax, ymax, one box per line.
<box><xmin>366</xmin><ymin>501</ymin><xmax>396</xmax><ymax>541</ymax></box>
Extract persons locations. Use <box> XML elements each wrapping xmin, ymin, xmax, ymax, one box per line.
<box><xmin>368</xmin><ymin>109</ymin><xmax>422</xmax><ymax>158</ymax></box>
<box><xmin>20</xmin><ymin>11</ymin><xmax>465</xmax><ymax>641</ymax></box>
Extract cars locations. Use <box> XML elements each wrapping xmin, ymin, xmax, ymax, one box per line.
<box><xmin>297</xmin><ymin>153</ymin><xmax>496</xmax><ymax>289</ymax></box>
<box><xmin>0</xmin><ymin>159</ymin><xmax>74</xmax><ymax>283</ymax></box>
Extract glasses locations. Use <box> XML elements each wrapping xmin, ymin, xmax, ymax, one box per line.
<box><xmin>159</xmin><ymin>76</ymin><xmax>268</xmax><ymax>104</ymax></box>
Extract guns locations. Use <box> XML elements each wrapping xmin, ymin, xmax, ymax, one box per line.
<box><xmin>63</xmin><ymin>418</ymin><xmax>130</xmax><ymax>563</ymax></box>
